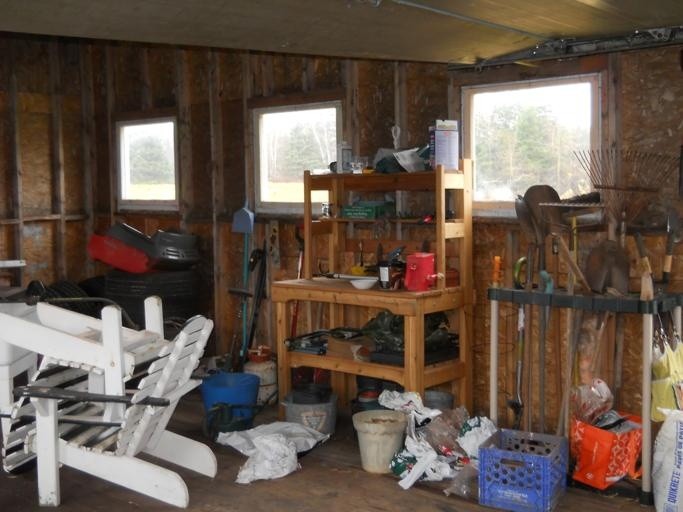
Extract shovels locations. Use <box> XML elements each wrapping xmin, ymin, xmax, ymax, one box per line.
<box><xmin>514</xmin><ymin>184</ymin><xmax>567</xmax><ymax>433</ymax></box>
<box><xmin>230</xmin><ymin>197</ymin><xmax>254</xmax><ymax>233</ymax></box>
<box><xmin>580</xmin><ymin>239</ymin><xmax>631</xmax><ymax>421</ymax></box>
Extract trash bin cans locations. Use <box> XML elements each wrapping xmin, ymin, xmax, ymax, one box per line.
<box><xmin>198</xmin><ymin>373</ymin><xmax>260</xmax><ymax>437</ymax></box>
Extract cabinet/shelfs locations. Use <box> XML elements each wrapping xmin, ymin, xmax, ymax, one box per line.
<box><xmin>268</xmin><ymin>158</ymin><xmax>476</xmax><ymax>426</ymax></box>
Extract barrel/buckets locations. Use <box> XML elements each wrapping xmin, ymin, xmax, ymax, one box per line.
<box><xmin>292</xmin><ymin>380</ymin><xmax>332</xmax><ymax>403</ymax></box>
<box><xmin>201</xmin><ymin>373</ymin><xmax>259</xmax><ymax>428</ymax></box>
<box><xmin>423</xmin><ymin>390</ymin><xmax>455</xmax><ymax>413</ymax></box>
<box><xmin>353</xmin><ymin>409</ymin><xmax>407</xmax><ymax>473</ymax></box>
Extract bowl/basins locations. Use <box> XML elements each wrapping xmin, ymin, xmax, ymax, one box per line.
<box><xmin>350</xmin><ymin>279</ymin><xmax>377</xmax><ymax>289</ymax></box>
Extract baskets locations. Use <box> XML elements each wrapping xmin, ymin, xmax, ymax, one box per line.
<box><xmin>475</xmin><ymin>426</ymin><xmax>569</xmax><ymax>512</ymax></box>
